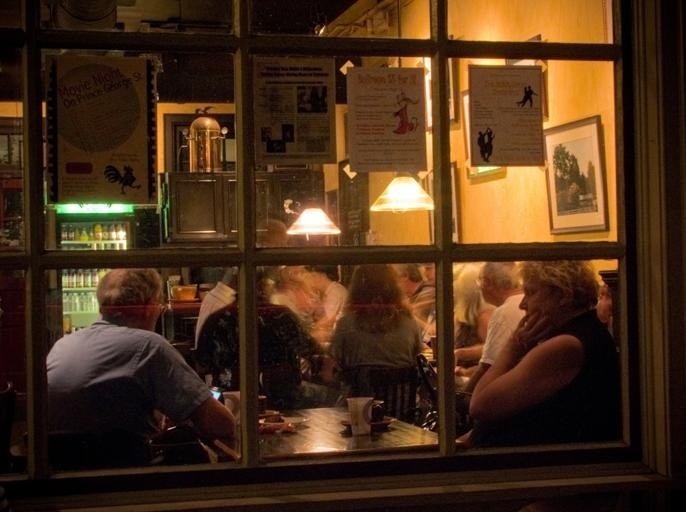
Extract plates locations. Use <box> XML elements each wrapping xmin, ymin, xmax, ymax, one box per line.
<box><xmin>340</xmin><ymin>416</ymin><xmax>396</xmax><ymax>429</ymax></box>
<box><xmin>166</xmin><ymin>298</ymin><xmax>199</xmax><ymax>303</ymax></box>
<box><xmin>259</xmin><ymin>416</ymin><xmax>310</xmax><ymax>427</ymax></box>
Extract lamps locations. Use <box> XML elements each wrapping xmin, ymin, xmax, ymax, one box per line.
<box><xmin>287</xmin><ymin>164</ymin><xmax>341</xmax><ymax>236</ymax></box>
<box><xmin>370</xmin><ymin>0</ymin><xmax>435</xmax><ymax>212</ymax></box>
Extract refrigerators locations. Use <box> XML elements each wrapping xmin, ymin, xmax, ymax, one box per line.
<box><xmin>43</xmin><ymin>204</ymin><xmax>176</xmax><ymax>352</ymax></box>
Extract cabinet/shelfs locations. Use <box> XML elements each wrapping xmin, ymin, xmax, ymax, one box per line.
<box><xmin>162</xmin><ymin>170</ymin><xmax>325</xmax><ymax>285</ymax></box>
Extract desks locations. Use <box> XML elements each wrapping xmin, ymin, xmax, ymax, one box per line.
<box><xmin>259</xmin><ymin>406</ymin><xmax>465</xmax><ymax>461</ymax></box>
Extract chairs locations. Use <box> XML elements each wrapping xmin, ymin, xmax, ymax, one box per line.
<box><xmin>0</xmin><ymin>380</ymin><xmax>15</xmax><ymax>465</ymax></box>
<box><xmin>260</xmin><ymin>354</ymin><xmax>438</xmax><ymax>421</ymax></box>
<box><xmin>22</xmin><ymin>430</ymin><xmax>149</xmax><ymax>471</ymax></box>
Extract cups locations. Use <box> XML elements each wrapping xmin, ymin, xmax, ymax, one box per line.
<box><xmin>371</xmin><ymin>400</ymin><xmax>386</xmax><ymax>422</ymax></box>
<box><xmin>346</xmin><ymin>396</ymin><xmax>375</xmax><ymax>437</ymax></box>
<box><xmin>170</xmin><ymin>285</ymin><xmax>197</xmax><ymax>299</ymax></box>
<box><xmin>222</xmin><ymin>390</ymin><xmax>241</xmax><ymax>426</ymax></box>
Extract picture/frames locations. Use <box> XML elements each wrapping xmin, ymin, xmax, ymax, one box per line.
<box><xmin>459</xmin><ymin>89</ymin><xmax>506</xmax><ymax>177</ymax></box>
<box><xmin>425</xmin><ymin>161</ymin><xmax>461</xmax><ymax>244</ymax></box>
<box><xmin>418</xmin><ymin>33</ymin><xmax>459</xmax><ymax>130</ymax></box>
<box><xmin>505</xmin><ymin>34</ymin><xmax>548</xmax><ymax>118</ymax></box>
<box><xmin>540</xmin><ymin>115</ymin><xmax>610</xmax><ymax>234</ymax></box>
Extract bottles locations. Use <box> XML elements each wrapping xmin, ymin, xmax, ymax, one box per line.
<box><xmin>62</xmin><ymin>291</ymin><xmax>98</xmax><ymax>311</ymax></box>
<box><xmin>61</xmin><ymin>267</ymin><xmax>106</xmax><ymax>289</ymax></box>
<box><xmin>59</xmin><ymin>221</ymin><xmax>127</xmax><ymax>250</ymax></box>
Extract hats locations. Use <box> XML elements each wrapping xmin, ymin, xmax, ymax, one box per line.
<box><xmin>226</xmin><ymin>264</ymin><xmax>281</xmax><ymax>292</ymax></box>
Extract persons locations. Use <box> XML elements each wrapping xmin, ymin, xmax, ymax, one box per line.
<box><xmin>196</xmin><ymin>218</ymin><xmax>623</xmax><ymax>452</ymax></box>
<box><xmin>393</xmin><ymin>92</ymin><xmax>419</xmax><ymax>133</ymax></box>
<box><xmin>43</xmin><ymin>266</ymin><xmax>238</xmax><ymax>471</ymax></box>
